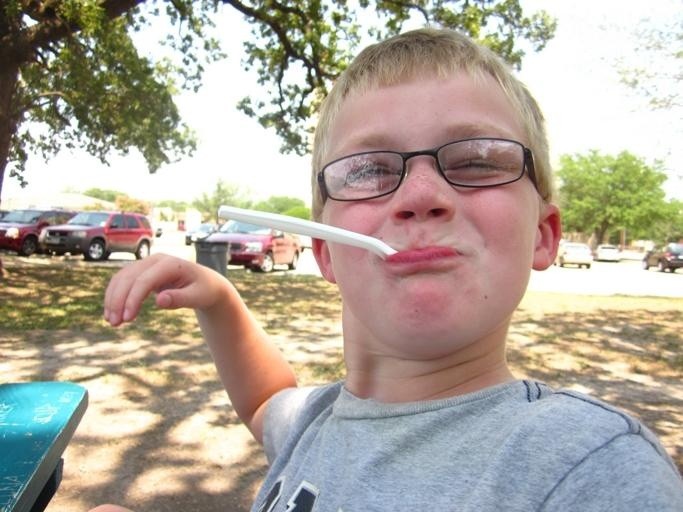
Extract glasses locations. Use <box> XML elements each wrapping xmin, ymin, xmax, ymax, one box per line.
<box><xmin>317</xmin><ymin>139</ymin><xmax>537</xmax><ymax>202</ymax></box>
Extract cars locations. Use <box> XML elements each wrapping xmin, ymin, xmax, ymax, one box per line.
<box><xmin>553</xmin><ymin>242</ymin><xmax>593</xmax><ymax>269</ymax></box>
<box><xmin>0</xmin><ymin>210</ymin><xmax>79</xmax><ymax>256</ymax></box>
<box><xmin>592</xmin><ymin>242</ymin><xmax>621</xmax><ymax>262</ymax></box>
<box><xmin>641</xmin><ymin>243</ymin><xmax>682</xmax><ymax>272</ymax></box>
<box><xmin>0</xmin><ymin>210</ymin><xmax>34</xmax><ymax>225</ymax></box>
<box><xmin>193</xmin><ymin>218</ymin><xmax>303</xmax><ymax>273</ymax></box>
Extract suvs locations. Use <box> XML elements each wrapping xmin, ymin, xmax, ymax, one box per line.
<box><xmin>37</xmin><ymin>210</ymin><xmax>153</xmax><ymax>262</ymax></box>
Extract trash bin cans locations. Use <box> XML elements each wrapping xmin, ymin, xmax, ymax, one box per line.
<box><xmin>195</xmin><ymin>240</ymin><xmax>230</xmax><ymax>277</ymax></box>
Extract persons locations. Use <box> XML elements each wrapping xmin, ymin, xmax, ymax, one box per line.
<box><xmin>104</xmin><ymin>29</ymin><xmax>683</xmax><ymax>510</ymax></box>
